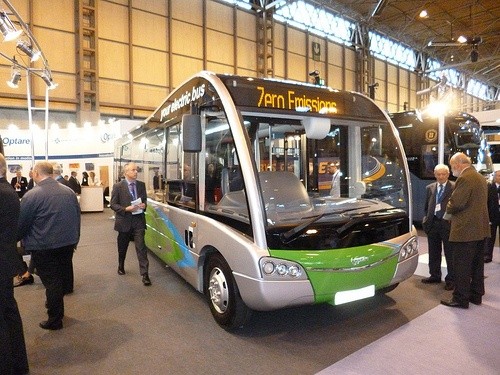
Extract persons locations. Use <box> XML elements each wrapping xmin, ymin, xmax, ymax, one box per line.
<box><xmin>10</xmin><ymin>170</ymin><xmax>34</xmax><ymax>199</ymax></box>
<box><xmin>109</xmin><ymin>161</ymin><xmax>155</xmax><ymax>288</ymax></box>
<box><xmin>437</xmin><ymin>151</ymin><xmax>490</xmax><ymax>308</ymax></box>
<box><xmin>0</xmin><ymin>152</ymin><xmax>35</xmax><ymax>375</ymax></box>
<box><xmin>478</xmin><ymin>165</ymin><xmax>500</xmax><ymax>265</ymax></box>
<box><xmin>421</xmin><ymin>160</ymin><xmax>461</xmax><ymax>290</ymax></box>
<box><xmin>12</xmin><ymin>239</ymin><xmax>79</xmax><ymax>294</ymax></box>
<box><xmin>51</xmin><ymin>162</ymin><xmax>101</xmax><ymax>196</ymax></box>
<box><xmin>19</xmin><ymin>161</ymin><xmax>84</xmax><ymax>332</ymax></box>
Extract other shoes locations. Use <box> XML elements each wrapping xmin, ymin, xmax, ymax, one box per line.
<box><xmin>441</xmin><ymin>298</ymin><xmax>468</xmax><ymax>308</ymax></box>
<box><xmin>422</xmin><ymin>277</ymin><xmax>440</xmax><ymax>283</ymax></box>
<box><xmin>445</xmin><ymin>283</ymin><xmax>455</xmax><ymax>290</ymax></box>
<box><xmin>142</xmin><ymin>277</ymin><xmax>151</xmax><ymax>285</ymax></box>
<box><xmin>39</xmin><ymin>321</ymin><xmax>53</xmax><ymax>329</ymax></box>
<box><xmin>118</xmin><ymin>264</ymin><xmax>125</xmax><ymax>274</ymax></box>
<box><xmin>471</xmin><ymin>298</ymin><xmax>483</xmax><ymax>305</ymax></box>
<box><xmin>14</xmin><ymin>275</ymin><xmax>34</xmax><ymax>287</ymax></box>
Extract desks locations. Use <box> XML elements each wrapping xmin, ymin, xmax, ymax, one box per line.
<box><xmin>80</xmin><ymin>185</ymin><xmax>103</xmax><ymax>213</ymax></box>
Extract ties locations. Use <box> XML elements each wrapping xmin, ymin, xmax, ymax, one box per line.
<box><xmin>438</xmin><ymin>186</ymin><xmax>443</xmax><ymax>198</ymax></box>
<box><xmin>131</xmin><ymin>183</ymin><xmax>136</xmax><ymax>200</ymax></box>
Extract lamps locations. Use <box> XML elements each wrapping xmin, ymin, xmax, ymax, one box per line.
<box><xmin>16</xmin><ymin>39</ymin><xmax>42</xmax><ymax>63</ymax></box>
<box><xmin>6</xmin><ymin>68</ymin><xmax>23</xmax><ymax>89</ymax></box>
<box><xmin>0</xmin><ymin>9</ymin><xmax>24</xmax><ymax>43</ymax></box>
<box><xmin>42</xmin><ymin>72</ymin><xmax>58</xmax><ymax>91</ymax></box>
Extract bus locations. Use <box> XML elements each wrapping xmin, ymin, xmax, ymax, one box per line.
<box><xmin>112</xmin><ymin>70</ymin><xmax>421</xmax><ymax>332</ymax></box>
<box><xmin>260</xmin><ymin>107</ymin><xmax>494</xmax><ymax>226</ymax></box>
<box><xmin>483</xmin><ymin>132</ymin><xmax>500</xmax><ymax>172</ymax></box>
